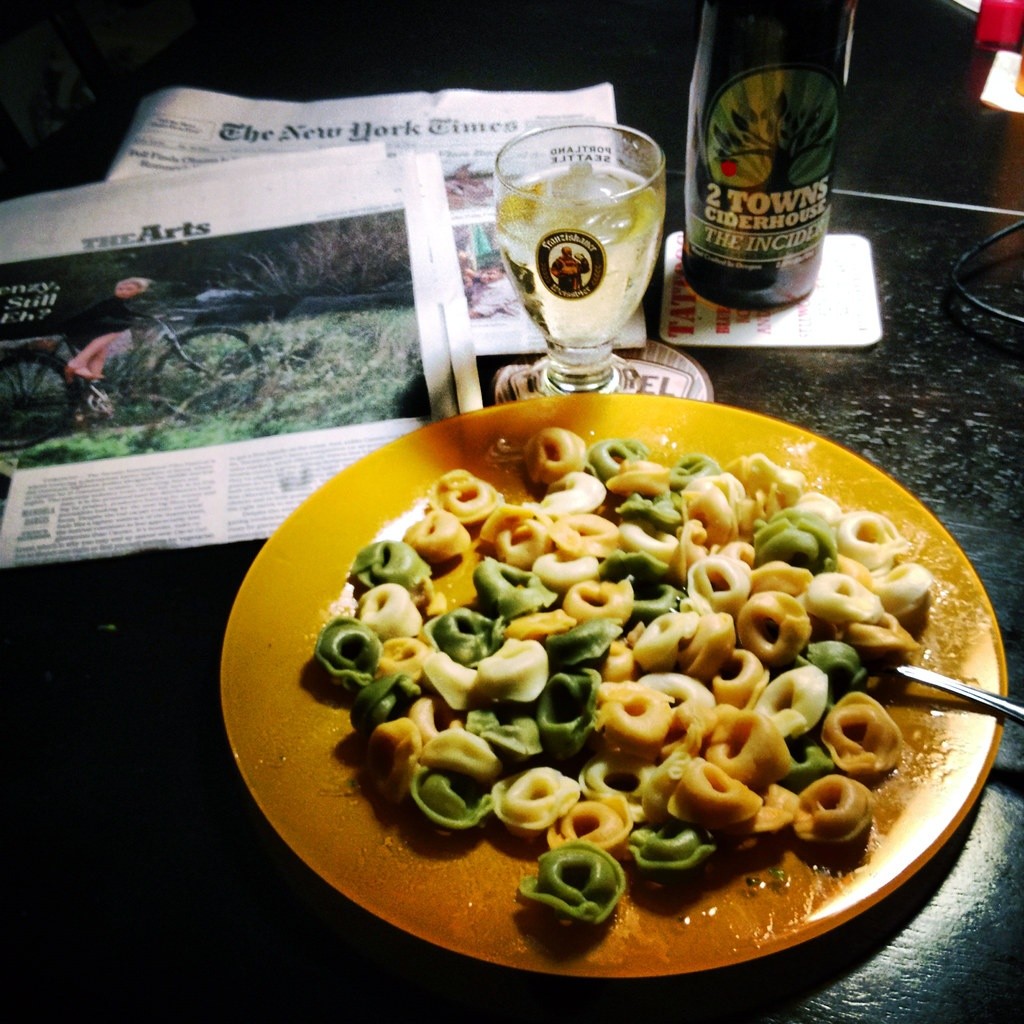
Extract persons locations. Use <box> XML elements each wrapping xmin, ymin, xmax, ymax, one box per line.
<box><xmin>551</xmin><ymin>247</ymin><xmax>590</xmax><ymax>290</ymax></box>
<box><xmin>59</xmin><ymin>279</ymin><xmax>152</xmax><ymax>376</ymax></box>
<box><xmin>446</xmin><ymin>232</ymin><xmax>522</xmax><ymax>319</ymax></box>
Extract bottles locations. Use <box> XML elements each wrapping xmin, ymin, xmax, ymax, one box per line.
<box><xmin>682</xmin><ymin>2</ymin><xmax>855</xmax><ymax>311</ymax></box>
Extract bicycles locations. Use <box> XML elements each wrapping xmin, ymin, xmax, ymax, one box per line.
<box><xmin>0</xmin><ymin>308</ymin><xmax>266</xmax><ymax>449</ymax></box>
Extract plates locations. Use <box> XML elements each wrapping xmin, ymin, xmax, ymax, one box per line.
<box><xmin>222</xmin><ymin>393</ymin><xmax>1009</xmax><ymax>981</ymax></box>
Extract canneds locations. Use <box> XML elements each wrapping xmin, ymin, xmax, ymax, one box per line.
<box><xmin>683</xmin><ymin>0</ymin><xmax>860</xmax><ymax>310</ymax></box>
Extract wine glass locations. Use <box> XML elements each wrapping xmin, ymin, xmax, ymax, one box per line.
<box><xmin>491</xmin><ymin>121</ymin><xmax>667</xmax><ymax>400</ymax></box>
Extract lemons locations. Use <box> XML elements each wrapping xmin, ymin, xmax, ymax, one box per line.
<box><xmin>500</xmin><ymin>173</ymin><xmax>657</xmax><ymax>247</ymax></box>
